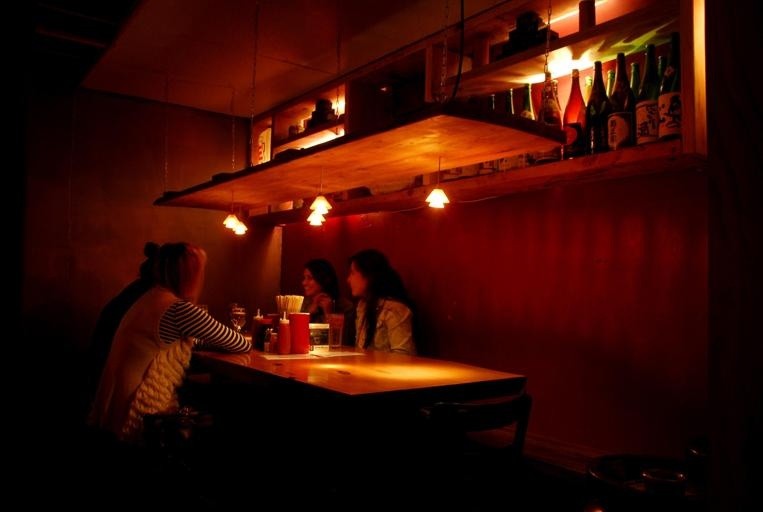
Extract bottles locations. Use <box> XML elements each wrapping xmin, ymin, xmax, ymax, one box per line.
<box><xmin>539</xmin><ymin>72</ymin><xmax>562</xmax><ymax>129</ymax></box>
<box><xmin>563</xmin><ymin>68</ymin><xmax>589</xmax><ymax>160</ymax></box>
<box><xmin>257</xmin><ymin>319</ymin><xmax>272</xmax><ymax>351</ymax></box>
<box><xmin>520</xmin><ymin>81</ymin><xmax>535</xmax><ymax>165</ymax></box>
<box><xmin>270</xmin><ymin>332</ymin><xmax>278</xmax><ymax>352</ymax></box>
<box><xmin>586</xmin><ymin>33</ymin><xmax>682</xmax><ymax>154</ymax></box>
<box><xmin>442</xmin><ymin>88</ymin><xmax>515</xmax><ymax>178</ymax></box>
<box><xmin>278</xmin><ymin>312</ymin><xmax>291</xmax><ymax>355</ymax></box>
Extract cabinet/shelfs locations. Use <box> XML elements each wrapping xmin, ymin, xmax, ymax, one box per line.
<box><xmin>247</xmin><ymin>42</ymin><xmax>431</xmax><ymax>224</ymax></box>
<box><xmin>431</xmin><ymin>0</ymin><xmax>709</xmax><ymax>203</ymax></box>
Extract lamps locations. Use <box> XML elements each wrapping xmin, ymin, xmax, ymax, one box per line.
<box><xmin>232</xmin><ymin>203</ymin><xmax>248</xmax><ymax>236</ymax></box>
<box><xmin>425</xmin><ymin>156</ymin><xmax>450</xmax><ymax>210</ymax></box>
<box><xmin>307</xmin><ymin>185</ymin><xmax>327</xmax><ymax>226</ymax></box>
<box><xmin>309</xmin><ymin>167</ymin><xmax>332</xmax><ymax>215</ymax></box>
<box><xmin>223</xmin><ymin>190</ymin><xmax>240</xmax><ymax>231</ymax></box>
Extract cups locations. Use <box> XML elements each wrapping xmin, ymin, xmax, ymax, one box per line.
<box><xmin>327</xmin><ymin>313</ymin><xmax>344</xmax><ymax>348</ymax></box>
<box><xmin>289</xmin><ymin>313</ymin><xmax>310</xmax><ymax>354</ymax></box>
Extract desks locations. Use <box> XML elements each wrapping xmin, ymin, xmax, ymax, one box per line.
<box><xmin>183</xmin><ymin>330</ymin><xmax>527</xmax><ymax>399</ymax></box>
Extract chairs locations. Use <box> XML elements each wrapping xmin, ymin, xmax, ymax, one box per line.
<box><xmin>422</xmin><ymin>395</ymin><xmax>532</xmax><ymax>505</ymax></box>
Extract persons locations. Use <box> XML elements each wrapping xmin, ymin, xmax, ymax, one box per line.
<box><xmin>343</xmin><ymin>248</ymin><xmax>418</xmax><ymax>356</ymax></box>
<box><xmin>87</xmin><ymin>242</ymin><xmax>252</xmax><ymax>463</ymax></box>
<box><xmin>91</xmin><ymin>240</ymin><xmax>215</xmax><ymax>412</ymax></box>
<box><xmin>299</xmin><ymin>257</ymin><xmax>339</xmax><ymax>324</ymax></box>
